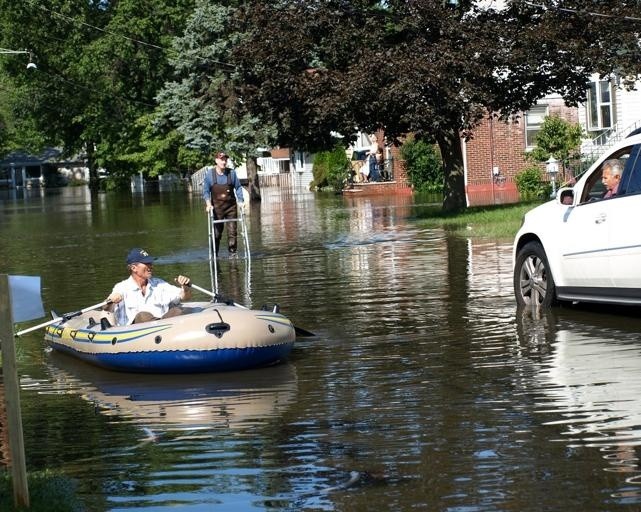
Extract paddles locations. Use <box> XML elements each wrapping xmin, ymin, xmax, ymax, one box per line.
<box><xmin>178</xmin><ymin>277</ymin><xmax>316</xmax><ymax>336</ymax></box>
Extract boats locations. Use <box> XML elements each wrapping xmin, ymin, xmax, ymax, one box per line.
<box><xmin>44</xmin><ymin>300</ymin><xmax>295</xmax><ymax>374</ymax></box>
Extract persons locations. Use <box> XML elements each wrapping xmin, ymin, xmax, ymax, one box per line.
<box><xmin>103</xmin><ymin>247</ymin><xmax>193</xmax><ymax>326</ymax></box>
<box><xmin>599</xmin><ymin>158</ymin><xmax>626</xmax><ymax>201</ymax></box>
<box><xmin>562</xmin><ymin>196</ymin><xmax>573</xmax><ymax>205</ymax></box>
<box><xmin>203</xmin><ymin>153</ymin><xmax>247</xmax><ymax>259</ymax></box>
<box><xmin>358</xmin><ymin>134</ymin><xmax>393</xmax><ymax>182</ymax></box>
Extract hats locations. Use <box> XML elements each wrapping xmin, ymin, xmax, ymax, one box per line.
<box><xmin>214</xmin><ymin>152</ymin><xmax>229</xmax><ymax>159</ymax></box>
<box><xmin>126</xmin><ymin>247</ymin><xmax>157</xmax><ymax>264</ymax></box>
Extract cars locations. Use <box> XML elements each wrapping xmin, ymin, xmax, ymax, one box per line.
<box><xmin>512</xmin><ymin>128</ymin><xmax>640</xmax><ymax>310</ymax></box>
